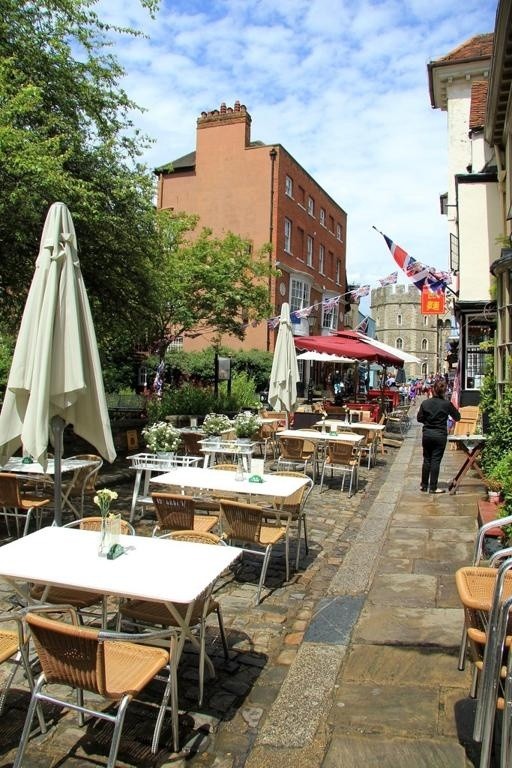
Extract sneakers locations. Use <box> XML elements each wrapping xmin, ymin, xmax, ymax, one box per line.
<box><xmin>429</xmin><ymin>487</ymin><xmax>446</xmax><ymax>494</ymax></box>
<box><xmin>420</xmin><ymin>485</ymin><xmax>427</xmax><ymax>492</ymax></box>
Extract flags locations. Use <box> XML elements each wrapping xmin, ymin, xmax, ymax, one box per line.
<box><xmin>381</xmin><ymin>232</ymin><xmax>451</xmax><ymax>294</ymax></box>
<box><xmin>267</xmin><ymin>271</ymin><xmax>398</xmax><ymax>330</ymax></box>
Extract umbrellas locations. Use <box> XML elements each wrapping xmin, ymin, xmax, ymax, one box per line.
<box><xmin>293</xmin><ymin>329</ymin><xmax>423</xmax><ymax>403</ymax></box>
<box><xmin>267</xmin><ymin>301</ymin><xmax>303</xmax><ymax>431</ymax></box>
<box><xmin>0</xmin><ymin>198</ymin><xmax>119</xmax><ymax>530</ymax></box>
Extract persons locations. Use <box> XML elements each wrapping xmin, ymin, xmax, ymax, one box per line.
<box><xmin>415</xmin><ymin>381</ymin><xmax>461</xmax><ymax>494</ymax></box>
<box><xmin>380</xmin><ymin>371</ymin><xmax>444</xmax><ymax>405</ymax></box>
